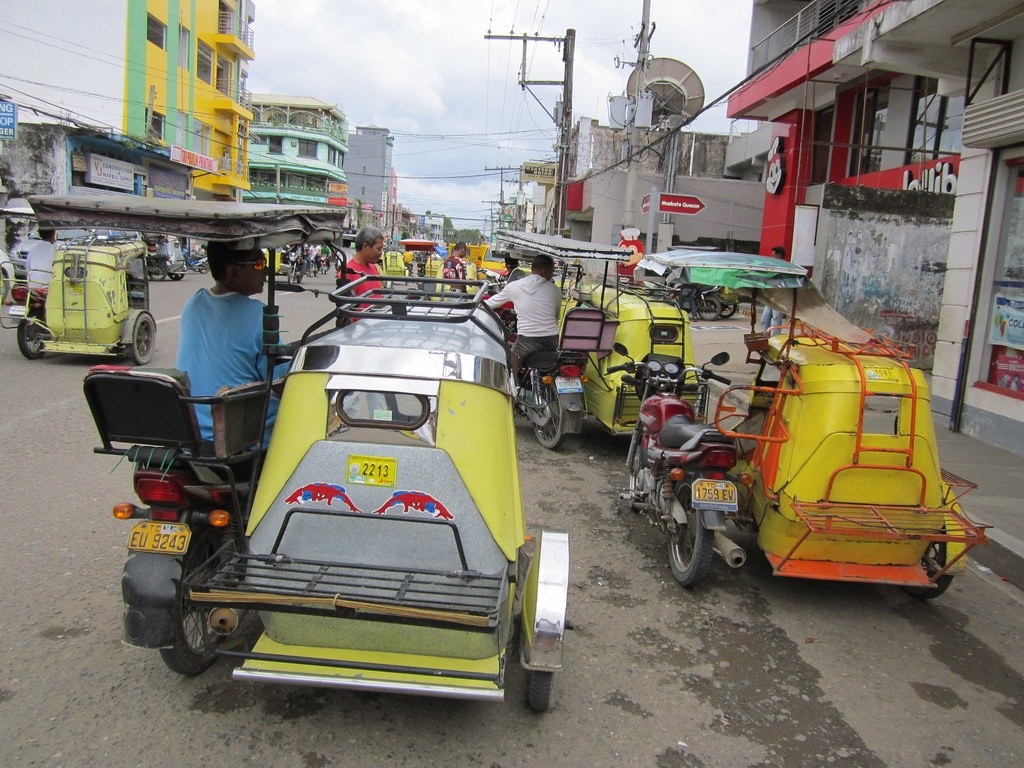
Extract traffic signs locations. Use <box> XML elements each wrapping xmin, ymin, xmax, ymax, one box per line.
<box><xmin>639</xmin><ymin>193</ymin><xmax>650</xmax><ymax>214</ymax></box>
<box><xmin>658</xmin><ymin>193</ymin><xmax>706</xmax><ymax>215</ymax></box>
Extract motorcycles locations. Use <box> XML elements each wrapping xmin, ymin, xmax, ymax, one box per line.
<box><xmin>22</xmin><ymin>187</ymin><xmax>572</xmax><ymax>715</ymax></box>
<box><xmin>603</xmin><ymin>332</ymin><xmax>995</xmax><ymax>600</ymax></box>
<box><xmin>0</xmin><ymin>229</ymin><xmax>699</xmax><ymax>449</ymax></box>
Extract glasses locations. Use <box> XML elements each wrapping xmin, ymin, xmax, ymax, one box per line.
<box><xmin>234</xmin><ymin>258</ymin><xmax>267</xmax><ymax>270</ymax></box>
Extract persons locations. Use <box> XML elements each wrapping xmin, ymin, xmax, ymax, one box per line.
<box><xmin>25</xmin><ymin>226</ymin><xmax>59</xmax><ymax>289</ymax></box>
<box><xmin>442</xmin><ymin>241</ymin><xmax>519</xmax><ymax>293</ymax></box>
<box><xmin>760</xmin><ymin>246</ymin><xmax>785</xmax><ymax>339</ymax></box>
<box><xmin>385</xmin><ymin>222</ymin><xmax>441</xmax><ymax>253</ymax></box>
<box><xmin>474</xmin><ymin>251</ymin><xmax>562</xmax><ymax>392</ymax></box>
<box><xmin>280</xmin><ymin>241</ymin><xmax>335</xmax><ymax>283</ymax></box>
<box><xmin>176</xmin><ymin>235</ymin><xmax>292</xmax><ymax>452</ymax></box>
<box><xmin>336</xmin><ymin>227</ymin><xmax>384</xmax><ymax>324</ymax></box>
<box><xmin>402</xmin><ymin>247</ymin><xmax>416</xmax><ymax>273</ymax></box>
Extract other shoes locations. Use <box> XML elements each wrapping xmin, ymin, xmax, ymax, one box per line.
<box><xmin>693</xmin><ymin>315</ymin><xmax>697</xmax><ymax>322</ymax></box>
